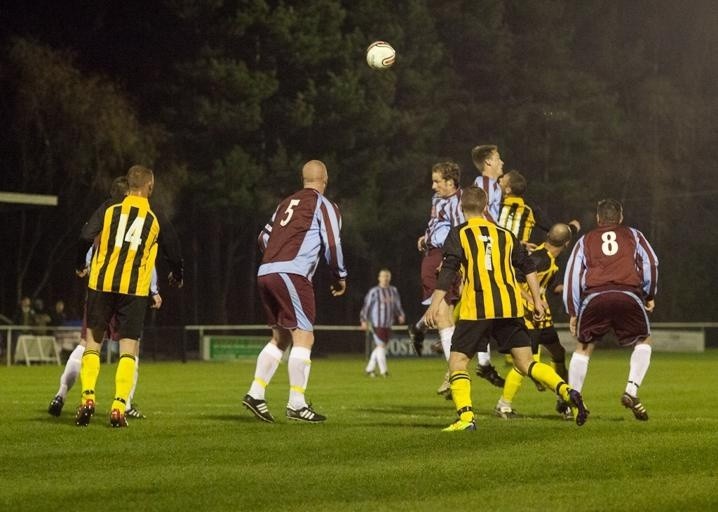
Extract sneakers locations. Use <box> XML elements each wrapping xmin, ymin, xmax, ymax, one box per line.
<box><xmin>76</xmin><ymin>399</ymin><xmax>95</xmax><ymax>426</ymax></box>
<box><xmin>495</xmin><ymin>406</ymin><xmax>519</xmax><ymax>418</ymax></box>
<box><xmin>110</xmin><ymin>409</ymin><xmax>143</xmax><ymax>427</ymax></box>
<box><xmin>441</xmin><ymin>417</ymin><xmax>476</xmax><ymax>432</ymax></box>
<box><xmin>476</xmin><ymin>363</ymin><xmax>505</xmax><ymax>387</ymax></box>
<box><xmin>50</xmin><ymin>396</ymin><xmax>64</xmax><ymax>416</ymax></box>
<box><xmin>622</xmin><ymin>392</ymin><xmax>648</xmax><ymax>420</ymax></box>
<box><xmin>242</xmin><ymin>394</ymin><xmax>274</xmax><ymax>423</ymax></box>
<box><xmin>556</xmin><ymin>388</ymin><xmax>589</xmax><ymax>426</ymax></box>
<box><xmin>286</xmin><ymin>399</ymin><xmax>325</xmax><ymax>421</ymax></box>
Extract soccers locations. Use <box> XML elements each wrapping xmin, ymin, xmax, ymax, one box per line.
<box><xmin>366</xmin><ymin>41</ymin><xmax>396</xmax><ymax>69</ymax></box>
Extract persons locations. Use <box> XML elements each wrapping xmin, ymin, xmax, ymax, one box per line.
<box><xmin>563</xmin><ymin>198</ymin><xmax>660</xmax><ymax>421</ymax></box>
<box><xmin>48</xmin><ymin>175</ymin><xmax>162</xmax><ymax>418</ymax></box>
<box><xmin>408</xmin><ymin>143</ymin><xmax>589</xmax><ymax>434</ymax></box>
<box><xmin>73</xmin><ymin>164</ymin><xmax>183</xmax><ymax>427</ymax></box>
<box><xmin>359</xmin><ymin>267</ymin><xmax>406</xmax><ymax>379</ymax></box>
<box><xmin>14</xmin><ymin>296</ymin><xmax>66</xmax><ymax>337</ymax></box>
<box><xmin>242</xmin><ymin>159</ymin><xmax>348</xmax><ymax>424</ymax></box>
